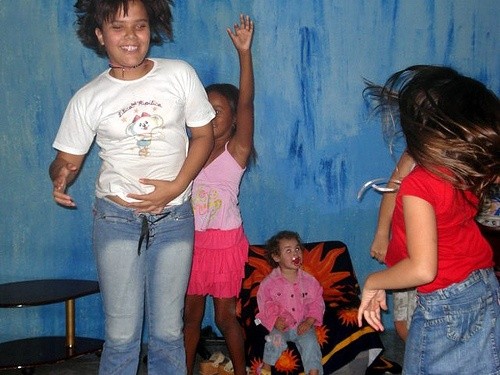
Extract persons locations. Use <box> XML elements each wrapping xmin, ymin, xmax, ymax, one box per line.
<box><xmin>356</xmin><ymin>64</ymin><xmax>500</xmax><ymax>375</ymax></box>
<box><xmin>256</xmin><ymin>231</ymin><xmax>325</xmax><ymax>375</ymax></box>
<box><xmin>182</xmin><ymin>12</ymin><xmax>255</xmax><ymax>375</ymax></box>
<box><xmin>49</xmin><ymin>0</ymin><xmax>217</xmax><ymax>375</ymax></box>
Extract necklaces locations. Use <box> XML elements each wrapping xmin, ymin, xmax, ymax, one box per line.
<box><xmin>109</xmin><ymin>57</ymin><xmax>145</xmax><ymax>77</ymax></box>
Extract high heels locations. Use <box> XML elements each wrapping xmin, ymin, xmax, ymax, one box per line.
<box><xmin>199</xmin><ymin>352</ymin><xmax>234</xmax><ymax>375</ymax></box>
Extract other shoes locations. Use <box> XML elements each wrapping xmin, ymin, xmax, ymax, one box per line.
<box><xmin>260</xmin><ymin>368</ymin><xmax>272</xmax><ymax>375</ymax></box>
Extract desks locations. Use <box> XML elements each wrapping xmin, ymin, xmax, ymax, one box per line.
<box><xmin>0</xmin><ymin>280</ymin><xmax>106</xmax><ymax>375</ymax></box>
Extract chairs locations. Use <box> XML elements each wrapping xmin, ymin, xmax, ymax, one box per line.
<box><xmin>235</xmin><ymin>240</ymin><xmax>402</xmax><ymax>375</ymax></box>
